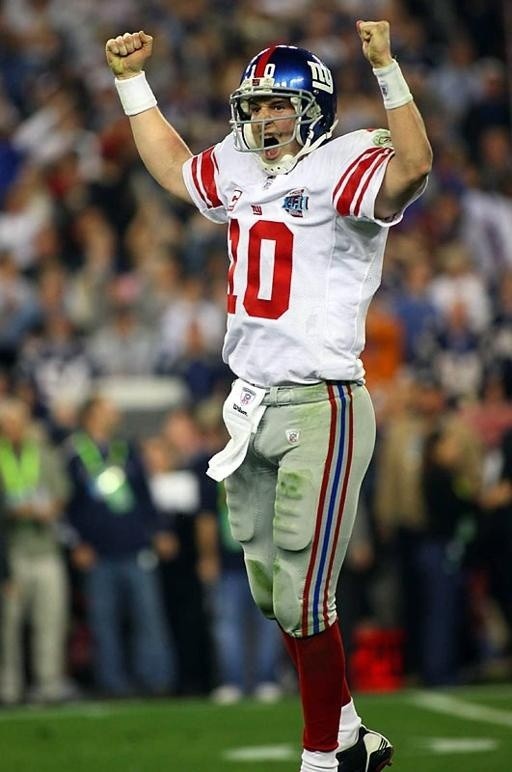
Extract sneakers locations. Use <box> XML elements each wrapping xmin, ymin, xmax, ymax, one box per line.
<box><xmin>336</xmin><ymin>724</ymin><xmax>396</xmax><ymax>772</ymax></box>
<box><xmin>212</xmin><ymin>682</ymin><xmax>284</xmax><ymax>706</ymax></box>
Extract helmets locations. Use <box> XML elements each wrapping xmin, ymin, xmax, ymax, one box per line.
<box><xmin>240</xmin><ymin>45</ymin><xmax>338</xmax><ymax>149</ymax></box>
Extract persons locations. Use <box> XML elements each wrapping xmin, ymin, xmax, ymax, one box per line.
<box><xmin>0</xmin><ymin>399</ymin><xmax>82</xmax><ymax>704</ymax></box>
<box><xmin>0</xmin><ymin>0</ymin><xmax>511</xmax><ymax>693</ymax></box>
<box><xmin>51</xmin><ymin>399</ymin><xmax>181</xmax><ymax>704</ymax></box>
<box><xmin>189</xmin><ymin>421</ymin><xmax>284</xmax><ymax>708</ymax></box>
<box><xmin>105</xmin><ymin>17</ymin><xmax>436</xmax><ymax>771</ymax></box>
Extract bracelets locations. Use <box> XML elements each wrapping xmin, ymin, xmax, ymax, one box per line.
<box><xmin>372</xmin><ymin>59</ymin><xmax>413</xmax><ymax>109</ymax></box>
<box><xmin>115</xmin><ymin>71</ymin><xmax>157</xmax><ymax>116</ymax></box>
